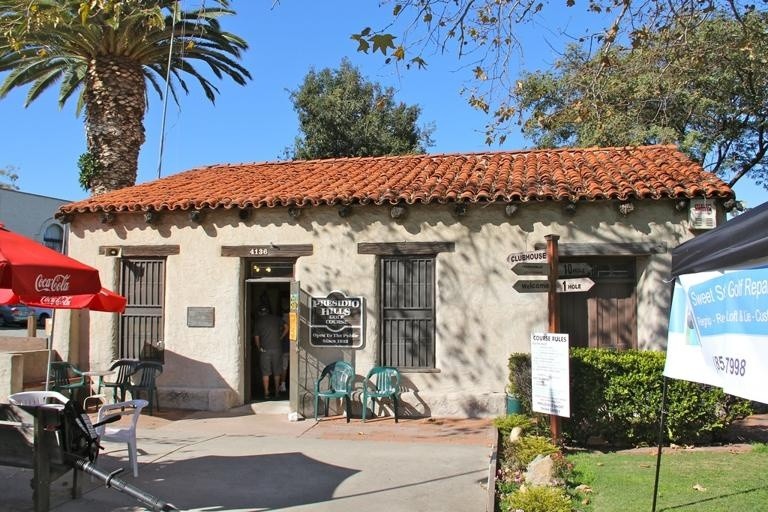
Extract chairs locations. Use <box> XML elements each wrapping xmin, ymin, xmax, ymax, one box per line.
<box><xmin>0</xmin><ymin>360</ymin><xmax>163</xmax><ymax>512</ymax></box>
<box><xmin>314</xmin><ymin>361</ymin><xmax>357</xmax><ymax>423</ymax></box>
<box><xmin>362</xmin><ymin>366</ymin><xmax>401</xmax><ymax>424</ymax></box>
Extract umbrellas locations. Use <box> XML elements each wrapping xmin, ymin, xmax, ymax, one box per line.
<box><xmin>0</xmin><ymin>286</ymin><xmax>128</xmax><ymax>402</ymax></box>
<box><xmin>0</xmin><ymin>221</ymin><xmax>102</xmax><ymax>297</ymax></box>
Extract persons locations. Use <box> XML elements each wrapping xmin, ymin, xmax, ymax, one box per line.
<box><xmin>251</xmin><ymin>303</ymin><xmax>289</xmax><ymax>401</ymax></box>
<box><xmin>273</xmin><ymin>298</ymin><xmax>289</xmax><ymax>394</ymax></box>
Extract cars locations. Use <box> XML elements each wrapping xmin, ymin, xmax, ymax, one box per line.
<box><xmin>0</xmin><ymin>304</ymin><xmax>52</xmax><ymax>328</ymax></box>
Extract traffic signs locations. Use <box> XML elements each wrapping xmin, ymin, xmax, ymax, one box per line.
<box><xmin>507</xmin><ymin>249</ymin><xmax>596</xmax><ymax>293</ymax></box>
